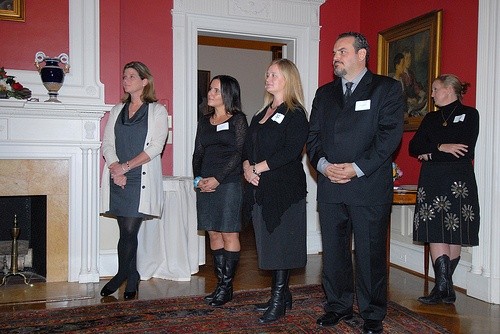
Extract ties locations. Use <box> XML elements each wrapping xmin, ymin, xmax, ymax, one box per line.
<box><xmin>344</xmin><ymin>82</ymin><xmax>354</xmax><ymax>102</ymax></box>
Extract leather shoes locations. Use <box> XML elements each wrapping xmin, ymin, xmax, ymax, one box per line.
<box><xmin>316</xmin><ymin>311</ymin><xmax>353</xmax><ymax>329</ymax></box>
<box><xmin>363</xmin><ymin>319</ymin><xmax>383</xmax><ymax>334</ymax></box>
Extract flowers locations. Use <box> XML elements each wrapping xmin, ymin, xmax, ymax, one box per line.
<box><xmin>392</xmin><ymin>162</ymin><xmax>403</xmax><ymax>182</ymax></box>
<box><xmin>0</xmin><ymin>67</ymin><xmax>32</xmax><ymax>99</ymax></box>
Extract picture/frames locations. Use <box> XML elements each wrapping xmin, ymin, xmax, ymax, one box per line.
<box><xmin>377</xmin><ymin>9</ymin><xmax>442</xmax><ymax>131</ymax></box>
<box><xmin>0</xmin><ymin>0</ymin><xmax>26</xmax><ymax>23</ymax></box>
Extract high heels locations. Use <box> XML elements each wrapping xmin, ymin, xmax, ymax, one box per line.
<box><xmin>99</xmin><ymin>274</ymin><xmax>127</xmax><ymax>297</ymax></box>
<box><xmin>123</xmin><ymin>271</ymin><xmax>140</xmax><ymax>299</ymax></box>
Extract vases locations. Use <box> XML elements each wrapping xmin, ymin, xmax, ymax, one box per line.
<box><xmin>35</xmin><ymin>52</ymin><xmax>70</xmax><ymax>103</ymax></box>
<box><xmin>0</xmin><ymin>99</ymin><xmax>27</xmax><ymax>108</ymax></box>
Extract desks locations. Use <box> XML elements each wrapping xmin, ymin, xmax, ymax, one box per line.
<box><xmin>136</xmin><ymin>176</ymin><xmax>206</xmax><ymax>282</ymax></box>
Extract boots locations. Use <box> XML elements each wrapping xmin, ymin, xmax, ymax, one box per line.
<box><xmin>417</xmin><ymin>254</ymin><xmax>461</xmax><ymax>305</ymax></box>
<box><xmin>254</xmin><ymin>268</ymin><xmax>293</xmax><ymax>324</ymax></box>
<box><xmin>203</xmin><ymin>248</ymin><xmax>240</xmax><ymax>307</ymax></box>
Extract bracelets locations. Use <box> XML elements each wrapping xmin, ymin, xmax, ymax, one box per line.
<box><xmin>438</xmin><ymin>144</ymin><xmax>441</xmax><ymax>151</ymax></box>
<box><xmin>253</xmin><ymin>162</ymin><xmax>261</xmax><ymax>176</ymax></box>
<box><xmin>194</xmin><ymin>176</ymin><xmax>203</xmax><ymax>189</ymax></box>
<box><xmin>428</xmin><ymin>153</ymin><xmax>431</xmax><ymax>162</ymax></box>
<box><xmin>126</xmin><ymin>161</ymin><xmax>131</xmax><ymax>171</ymax></box>
<box><xmin>122</xmin><ymin>163</ymin><xmax>127</xmax><ymax>173</ymax></box>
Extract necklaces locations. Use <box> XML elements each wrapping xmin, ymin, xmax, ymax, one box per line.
<box><xmin>441</xmin><ymin>105</ymin><xmax>457</xmax><ymax>126</ymax></box>
<box><xmin>213</xmin><ymin>114</ymin><xmax>231</xmax><ymax>124</ymax></box>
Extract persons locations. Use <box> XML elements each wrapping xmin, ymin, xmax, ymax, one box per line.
<box><xmin>192</xmin><ymin>76</ymin><xmax>246</xmax><ymax>306</ymax></box>
<box><xmin>100</xmin><ymin>61</ymin><xmax>169</xmax><ymax>300</ymax></box>
<box><xmin>306</xmin><ymin>33</ymin><xmax>404</xmax><ymax>334</ymax></box>
<box><xmin>241</xmin><ymin>59</ymin><xmax>309</xmax><ymax>324</ymax></box>
<box><xmin>409</xmin><ymin>74</ymin><xmax>479</xmax><ymax>305</ymax></box>
